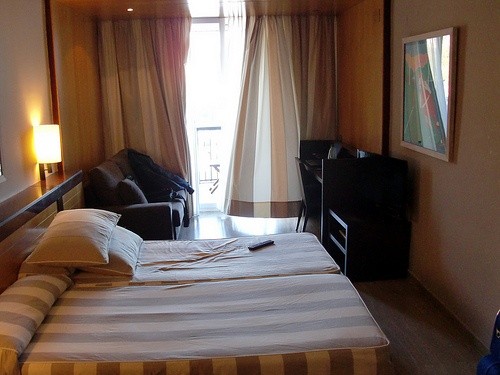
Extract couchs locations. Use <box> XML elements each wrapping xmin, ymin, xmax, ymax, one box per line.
<box><xmin>90</xmin><ymin>147</ymin><xmax>192</xmax><ymax>241</ymax></box>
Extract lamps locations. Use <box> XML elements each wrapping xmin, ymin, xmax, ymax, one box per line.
<box><xmin>34</xmin><ymin>125</ymin><xmax>62</xmax><ymax>172</ymax></box>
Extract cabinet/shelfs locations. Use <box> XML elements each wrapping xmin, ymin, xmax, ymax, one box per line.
<box><xmin>328</xmin><ymin>204</ymin><xmax>405</xmax><ymax>284</ymax></box>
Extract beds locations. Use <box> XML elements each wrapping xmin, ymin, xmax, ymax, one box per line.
<box><xmin>20</xmin><ymin>232</ymin><xmax>391</xmax><ymax>375</ymax></box>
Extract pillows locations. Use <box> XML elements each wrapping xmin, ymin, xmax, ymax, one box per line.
<box><xmin>80</xmin><ymin>226</ymin><xmax>145</xmax><ymax>275</ymax></box>
<box><xmin>24</xmin><ymin>208</ymin><xmax>120</xmax><ymax>268</ymax></box>
<box><xmin>0</xmin><ymin>274</ymin><xmax>72</xmax><ymax>375</ymax></box>
<box><xmin>19</xmin><ymin>259</ymin><xmax>75</xmax><ymax>278</ymax></box>
<box><xmin>118</xmin><ymin>178</ymin><xmax>148</xmax><ymax>204</ymax></box>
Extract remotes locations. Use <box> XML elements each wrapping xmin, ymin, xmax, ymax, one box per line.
<box><xmin>248</xmin><ymin>240</ymin><xmax>274</xmax><ymax>250</ymax></box>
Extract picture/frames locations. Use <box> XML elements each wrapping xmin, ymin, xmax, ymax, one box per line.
<box><xmin>399</xmin><ymin>26</ymin><xmax>459</xmax><ymax>161</ymax></box>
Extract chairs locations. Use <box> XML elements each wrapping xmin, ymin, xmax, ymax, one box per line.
<box><xmin>295</xmin><ymin>156</ymin><xmax>323</xmax><ymax>233</ymax></box>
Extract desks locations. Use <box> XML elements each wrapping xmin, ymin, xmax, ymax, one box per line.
<box><xmin>297</xmin><ymin>138</ymin><xmax>409</xmax><ymax>245</ymax></box>
